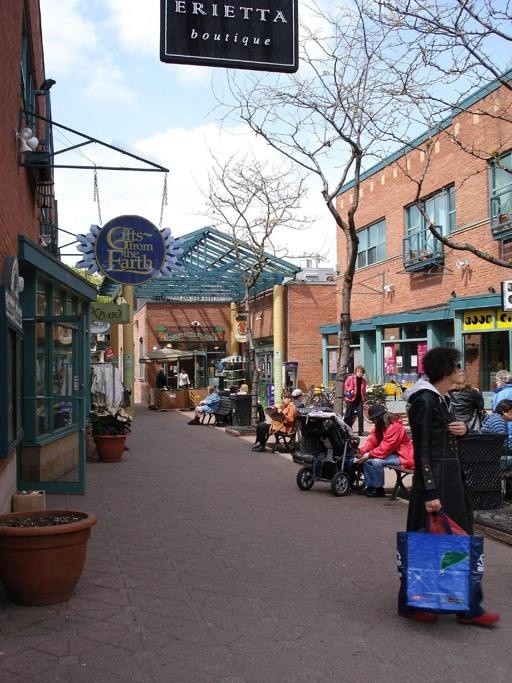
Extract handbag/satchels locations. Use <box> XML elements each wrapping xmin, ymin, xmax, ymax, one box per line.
<box><xmin>343</xmin><ymin>389</ymin><xmax>355</xmax><ymax>399</ymax></box>
<box><xmin>396</xmin><ymin>513</ymin><xmax>485</xmax><ymax>615</ymax></box>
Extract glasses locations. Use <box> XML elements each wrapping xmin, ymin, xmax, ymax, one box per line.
<box><xmin>455</xmin><ymin>363</ymin><xmax>461</xmax><ymax>369</ymax></box>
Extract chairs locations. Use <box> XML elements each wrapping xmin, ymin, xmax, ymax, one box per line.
<box><xmin>272</xmin><ymin>423</ymin><xmax>300</xmax><ymax>454</ymax></box>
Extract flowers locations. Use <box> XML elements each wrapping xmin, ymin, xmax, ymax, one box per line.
<box><xmin>89</xmin><ymin>404</ymin><xmax>133</xmax><ymax>435</ymax></box>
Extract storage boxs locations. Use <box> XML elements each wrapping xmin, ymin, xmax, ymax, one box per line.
<box><xmin>10</xmin><ymin>487</ymin><xmax>48</xmax><ymax>511</ymax></box>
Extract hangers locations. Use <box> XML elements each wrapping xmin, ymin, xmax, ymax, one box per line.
<box><xmin>90</xmin><ymin>359</ymin><xmax>114</xmax><ymax>371</ymax></box>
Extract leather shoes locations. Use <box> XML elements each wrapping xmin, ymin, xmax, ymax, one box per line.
<box><xmin>358</xmin><ymin>432</ymin><xmax>368</xmax><ymax>436</ymax></box>
<box><xmin>366</xmin><ymin>487</ymin><xmax>385</xmax><ymax>497</ymax></box>
<box><xmin>357</xmin><ymin>485</ymin><xmax>370</xmax><ymax>495</ymax></box>
<box><xmin>252</xmin><ymin>445</ymin><xmax>265</xmax><ymax>452</ymax></box>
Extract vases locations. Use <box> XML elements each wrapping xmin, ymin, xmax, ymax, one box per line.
<box><xmin>0</xmin><ymin>508</ymin><xmax>102</xmax><ymax>608</ymax></box>
<box><xmin>93</xmin><ymin>434</ymin><xmax>127</xmax><ymax>463</ymax></box>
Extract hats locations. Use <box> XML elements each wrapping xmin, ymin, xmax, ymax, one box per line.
<box><xmin>292</xmin><ymin>389</ymin><xmax>302</xmax><ymax>396</ymax></box>
<box><xmin>368</xmin><ymin>404</ymin><xmax>387</xmax><ymax>420</ymax></box>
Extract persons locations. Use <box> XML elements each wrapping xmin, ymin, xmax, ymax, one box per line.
<box><xmin>186</xmin><ymin>384</ymin><xmax>306</xmax><ymax>452</ymax></box>
<box><xmin>340</xmin><ymin>365</ymin><xmax>368</xmax><ymax>437</ymax></box>
<box><xmin>444</xmin><ymin>370</ymin><xmax>512</xmax><ymax>474</ymax></box>
<box><xmin>166</xmin><ymin>370</ymin><xmax>177</xmax><ymax>389</ymax></box>
<box><xmin>395</xmin><ymin>345</ymin><xmax>499</xmax><ymax>627</ymax></box>
<box><xmin>352</xmin><ymin>404</ymin><xmax>414</xmax><ymax>498</ymax></box>
<box><xmin>155</xmin><ymin>368</ymin><xmax>166</xmax><ymax>389</ymax></box>
<box><xmin>178</xmin><ymin>368</ymin><xmax>190</xmax><ymax>389</ymax></box>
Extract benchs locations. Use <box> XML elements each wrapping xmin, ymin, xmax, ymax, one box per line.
<box><xmin>386</xmin><ymin>426</ymin><xmax>417</xmax><ymax>503</ymax></box>
<box><xmin>202</xmin><ymin>401</ymin><xmax>234</xmax><ymax>427</ymax></box>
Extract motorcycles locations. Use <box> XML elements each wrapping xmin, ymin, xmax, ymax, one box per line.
<box><xmin>391</xmin><ymin>379</ymin><xmax>407</xmax><ymax>400</ymax></box>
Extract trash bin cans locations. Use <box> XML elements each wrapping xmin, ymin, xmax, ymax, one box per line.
<box><xmin>455</xmin><ymin>434</ymin><xmax>507</xmax><ymax>509</ymax></box>
<box><xmin>231</xmin><ymin>395</ymin><xmax>251</xmax><ymax>427</ymax></box>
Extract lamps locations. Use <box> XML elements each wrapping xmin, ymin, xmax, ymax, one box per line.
<box><xmin>254</xmin><ymin>310</ymin><xmax>263</xmax><ymax>321</ymax></box>
<box><xmin>37</xmin><ymin>232</ymin><xmax>54</xmax><ymax>247</ymax></box>
<box><xmin>12</xmin><ymin>125</ymin><xmax>39</xmax><ymax>152</ymax></box>
<box><xmin>190</xmin><ymin>318</ymin><xmax>201</xmax><ymax>327</ymax></box>
<box><xmin>382</xmin><ymin>282</ymin><xmax>395</xmax><ymax>293</ymax></box>
<box><xmin>453</xmin><ymin>259</ymin><xmax>472</xmax><ymax>272</ymax></box>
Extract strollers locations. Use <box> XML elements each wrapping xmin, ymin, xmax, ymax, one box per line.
<box><xmin>293</xmin><ymin>411</ymin><xmax>367</xmax><ymax>496</ymax></box>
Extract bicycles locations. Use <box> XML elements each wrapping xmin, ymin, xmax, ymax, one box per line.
<box><xmin>303</xmin><ymin>384</ymin><xmax>381</xmax><ymax>423</ymax></box>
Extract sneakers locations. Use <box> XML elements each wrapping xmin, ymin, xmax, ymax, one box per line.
<box><xmin>456</xmin><ymin>612</ymin><xmax>501</xmax><ymax>624</ymax></box>
<box><xmin>397</xmin><ymin>610</ymin><xmax>438</xmax><ymax>623</ymax></box>
